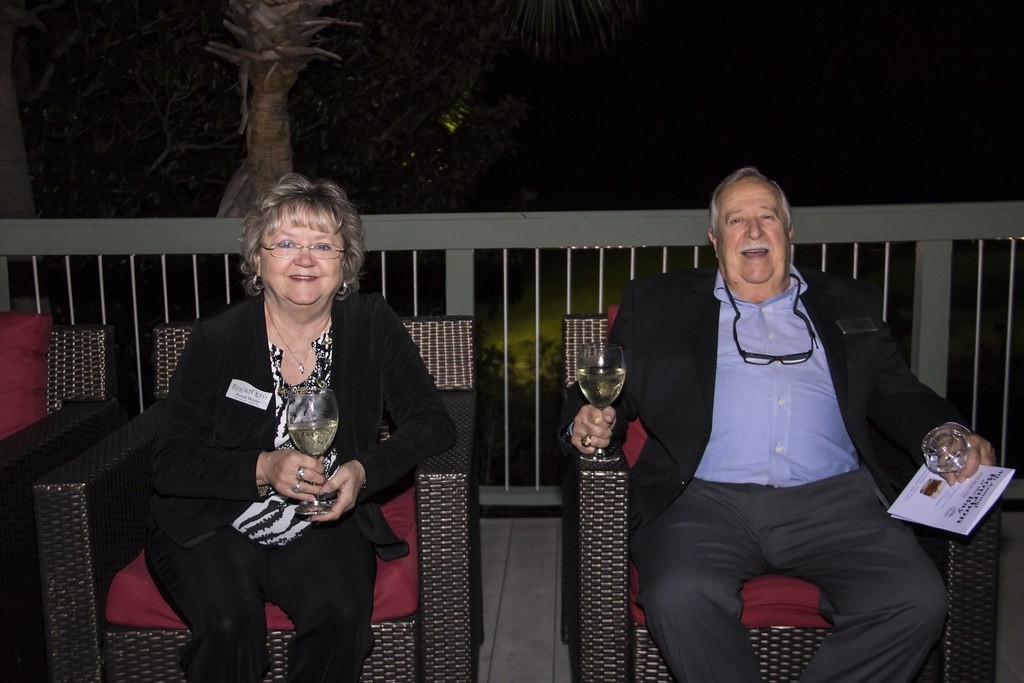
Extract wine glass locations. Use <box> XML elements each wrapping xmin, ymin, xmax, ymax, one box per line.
<box><xmin>577</xmin><ymin>342</ymin><xmax>626</xmax><ymax>462</ymax></box>
<box><xmin>285</xmin><ymin>387</ymin><xmax>339</xmax><ymax>516</ymax></box>
<box><xmin>921</xmin><ymin>427</ymin><xmax>972</xmax><ymax>479</ymax></box>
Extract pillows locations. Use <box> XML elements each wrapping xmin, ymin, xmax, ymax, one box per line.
<box><xmin>0</xmin><ymin>311</ymin><xmax>54</xmax><ymax>440</ymax></box>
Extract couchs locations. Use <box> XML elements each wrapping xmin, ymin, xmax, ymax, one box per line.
<box><xmin>33</xmin><ymin>314</ymin><xmax>486</xmax><ymax>683</ymax></box>
<box><xmin>559</xmin><ymin>313</ymin><xmax>1002</xmax><ymax>683</ymax></box>
<box><xmin>0</xmin><ymin>322</ymin><xmax>128</xmax><ymax>683</ymax></box>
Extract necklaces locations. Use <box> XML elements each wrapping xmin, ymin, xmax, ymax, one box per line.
<box><xmin>272</xmin><ymin>324</ymin><xmax>327</xmax><ymax>373</ymax></box>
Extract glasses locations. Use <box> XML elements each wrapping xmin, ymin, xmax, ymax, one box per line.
<box><xmin>733</xmin><ymin>309</ymin><xmax>813</xmax><ymax>365</ymax></box>
<box><xmin>257</xmin><ymin>236</ymin><xmax>348</xmax><ymax>261</ymax></box>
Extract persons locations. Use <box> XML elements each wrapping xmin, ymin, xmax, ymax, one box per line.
<box><xmin>145</xmin><ymin>175</ymin><xmax>456</xmax><ymax>683</ymax></box>
<box><xmin>556</xmin><ymin>168</ymin><xmax>996</xmax><ymax>683</ymax></box>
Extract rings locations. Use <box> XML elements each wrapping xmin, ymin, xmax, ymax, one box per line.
<box><xmin>292</xmin><ymin>481</ymin><xmax>301</xmax><ymax>493</ymax></box>
<box><xmin>581</xmin><ymin>435</ymin><xmax>591</xmax><ymax>446</ymax></box>
<box><xmin>296</xmin><ymin>467</ymin><xmax>305</xmax><ymax>480</ymax></box>
